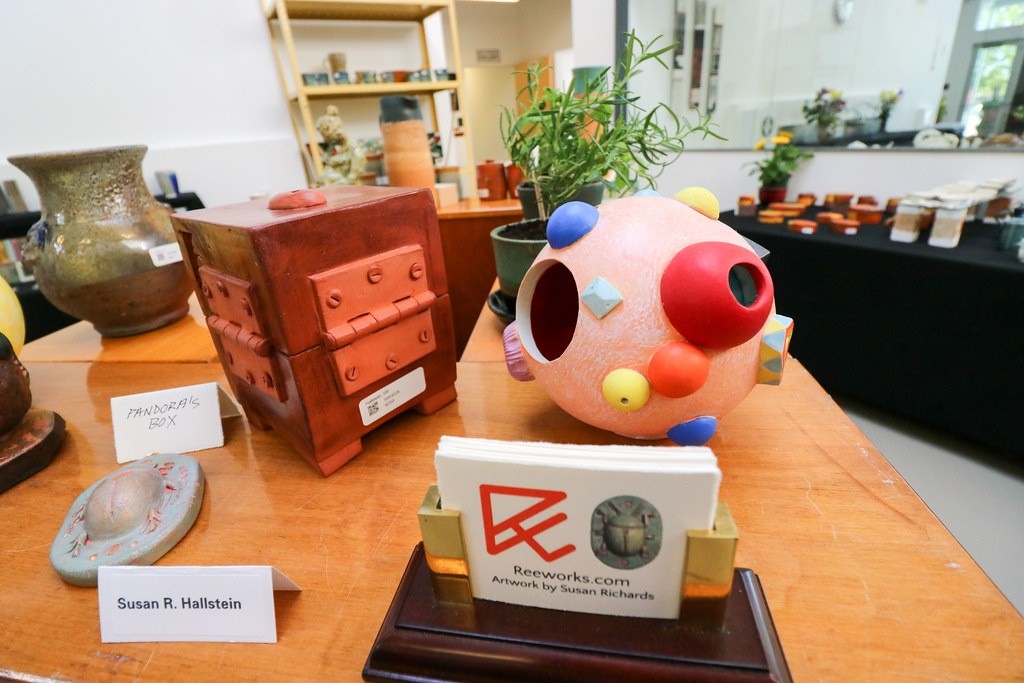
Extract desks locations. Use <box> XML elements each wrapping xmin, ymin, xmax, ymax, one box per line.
<box><xmin>0</xmin><ymin>358</ymin><xmax>1024</xmax><ymax>683</ymax></box>
<box><xmin>437</xmin><ymin>200</ymin><xmax>525</xmax><ymax>363</ymax></box>
<box><xmin>719</xmin><ymin>205</ymin><xmax>1024</xmax><ymax>480</ymax></box>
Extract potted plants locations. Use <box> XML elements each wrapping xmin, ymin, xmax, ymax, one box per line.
<box><xmin>485</xmin><ymin>28</ymin><xmax>730</xmax><ymax>325</ymax></box>
<box><xmin>516</xmin><ymin>104</ymin><xmax>604</xmax><ymax>220</ymax></box>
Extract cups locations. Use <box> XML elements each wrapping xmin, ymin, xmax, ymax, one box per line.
<box><xmin>332</xmin><ymin>71</ymin><xmax>348</xmax><ymax>85</ymax></box>
<box><xmin>435</xmin><ymin>70</ymin><xmax>447</xmax><ymax>80</ymax></box>
<box><xmin>998</xmin><ymin>217</ymin><xmax>1024</xmax><ymax>250</ymax></box>
<box><xmin>322</xmin><ymin>52</ymin><xmax>347</xmax><ymax>73</ymax></box>
<box><xmin>416</xmin><ymin>69</ymin><xmax>430</xmax><ymax>81</ymax></box>
<box><xmin>393</xmin><ymin>71</ymin><xmax>414</xmax><ymax>82</ymax></box>
<box><xmin>356</xmin><ymin>71</ymin><xmax>376</xmax><ymax>83</ymax></box>
<box><xmin>503</xmin><ymin>162</ymin><xmax>528</xmax><ymax>199</ymax></box>
<box><xmin>154</xmin><ymin>169</ymin><xmax>179</xmax><ymax>199</ymax></box>
<box><xmin>301</xmin><ymin>73</ymin><xmax>329</xmax><ymax>86</ymax></box>
<box><xmin>475</xmin><ymin>159</ymin><xmax>506</xmax><ymax>202</ymax></box>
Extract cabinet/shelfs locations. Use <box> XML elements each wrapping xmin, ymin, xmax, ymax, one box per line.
<box><xmin>259</xmin><ymin>0</ymin><xmax>476</xmax><ymax>190</ymax></box>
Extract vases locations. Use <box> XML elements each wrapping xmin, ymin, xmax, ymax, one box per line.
<box><xmin>6</xmin><ymin>144</ymin><xmax>192</xmax><ymax>338</ymax></box>
<box><xmin>759</xmin><ymin>187</ymin><xmax>787</xmax><ymax>204</ymax></box>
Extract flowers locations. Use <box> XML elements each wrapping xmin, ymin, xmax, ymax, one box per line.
<box><xmin>802</xmin><ymin>86</ymin><xmax>847</xmax><ymax>131</ymax></box>
<box><xmin>741</xmin><ymin>130</ymin><xmax>814</xmax><ymax>184</ymax></box>
<box><xmin>878</xmin><ymin>88</ymin><xmax>904</xmax><ymax>111</ymax></box>
